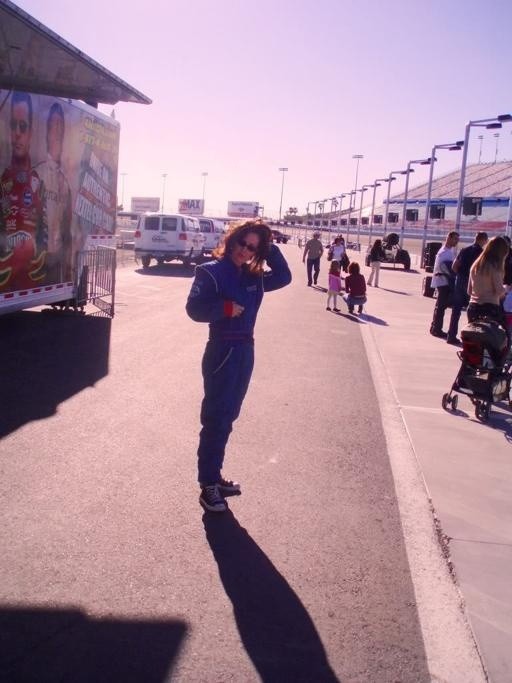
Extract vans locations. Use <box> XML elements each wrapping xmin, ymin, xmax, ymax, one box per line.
<box><xmin>134</xmin><ymin>212</ymin><xmax>206</xmax><ymax>271</ymax></box>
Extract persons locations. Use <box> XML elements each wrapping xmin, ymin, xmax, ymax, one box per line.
<box><xmin>467</xmin><ymin>236</ymin><xmax>512</xmax><ymax>329</ymax></box>
<box><xmin>303</xmin><ymin>231</ymin><xmax>324</xmax><ymax>287</ymax></box>
<box><xmin>502</xmin><ymin>235</ymin><xmax>512</xmax><ymax>314</ymax></box>
<box><xmin>328</xmin><ymin>236</ymin><xmax>345</xmax><ymax>269</ymax></box>
<box><xmin>429</xmin><ymin>231</ymin><xmax>459</xmax><ymax>340</ymax></box>
<box><xmin>326</xmin><ymin>258</ymin><xmax>341</xmax><ymax>312</ymax></box>
<box><xmin>0</xmin><ymin>91</ymin><xmax>48</xmax><ymax>294</ymax></box>
<box><xmin>32</xmin><ymin>101</ymin><xmax>73</xmax><ymax>284</ymax></box>
<box><xmin>447</xmin><ymin>231</ymin><xmax>489</xmax><ymax>345</ymax></box>
<box><xmin>343</xmin><ymin>262</ymin><xmax>367</xmax><ymax>314</ymax></box>
<box><xmin>185</xmin><ymin>216</ymin><xmax>291</xmax><ymax>512</ymax></box>
<box><xmin>367</xmin><ymin>240</ymin><xmax>384</xmax><ymax>289</ymax></box>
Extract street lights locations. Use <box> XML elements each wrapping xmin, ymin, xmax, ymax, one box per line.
<box><xmin>416</xmin><ymin>140</ymin><xmax>462</xmax><ymax>272</ymax></box>
<box><xmin>195</xmin><ymin>218</ymin><xmax>224</xmax><ymax>256</ymax></box>
<box><xmin>299</xmin><ymin>153</ymin><xmax>437</xmax><ymax>256</ymax></box>
<box><xmin>475</xmin><ymin>134</ymin><xmax>483</xmax><ymax>163</ymax></box>
<box><xmin>275</xmin><ymin>165</ymin><xmax>289</xmax><ymax>223</ymax></box>
<box><xmin>492</xmin><ymin>131</ymin><xmax>500</xmax><ymax>164</ymax></box>
<box><xmin>447</xmin><ymin>114</ymin><xmax>512</xmax><ymax>259</ymax></box>
<box><xmin>119</xmin><ymin>172</ymin><xmax>128</xmax><ymax>211</ymax></box>
<box><xmin>161</xmin><ymin>172</ymin><xmax>168</xmax><ymax>215</ymax></box>
<box><xmin>200</xmin><ymin>172</ymin><xmax>208</xmax><ymax>199</ymax></box>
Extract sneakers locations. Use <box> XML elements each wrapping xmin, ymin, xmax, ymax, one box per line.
<box><xmin>215</xmin><ymin>478</ymin><xmax>241</xmax><ymax>492</ymax></box>
<box><xmin>200</xmin><ymin>486</ymin><xmax>227</xmax><ymax>512</ymax></box>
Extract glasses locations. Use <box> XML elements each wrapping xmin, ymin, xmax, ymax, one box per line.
<box><xmin>236</xmin><ymin>238</ymin><xmax>255</xmax><ymax>252</ymax></box>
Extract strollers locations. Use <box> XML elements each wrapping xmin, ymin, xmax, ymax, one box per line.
<box><xmin>442</xmin><ymin>317</ymin><xmax>512</xmax><ymax>421</ymax></box>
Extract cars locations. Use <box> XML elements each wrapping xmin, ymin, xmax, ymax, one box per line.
<box><xmin>272</xmin><ymin>229</ymin><xmax>291</xmax><ymax>240</ymax></box>
